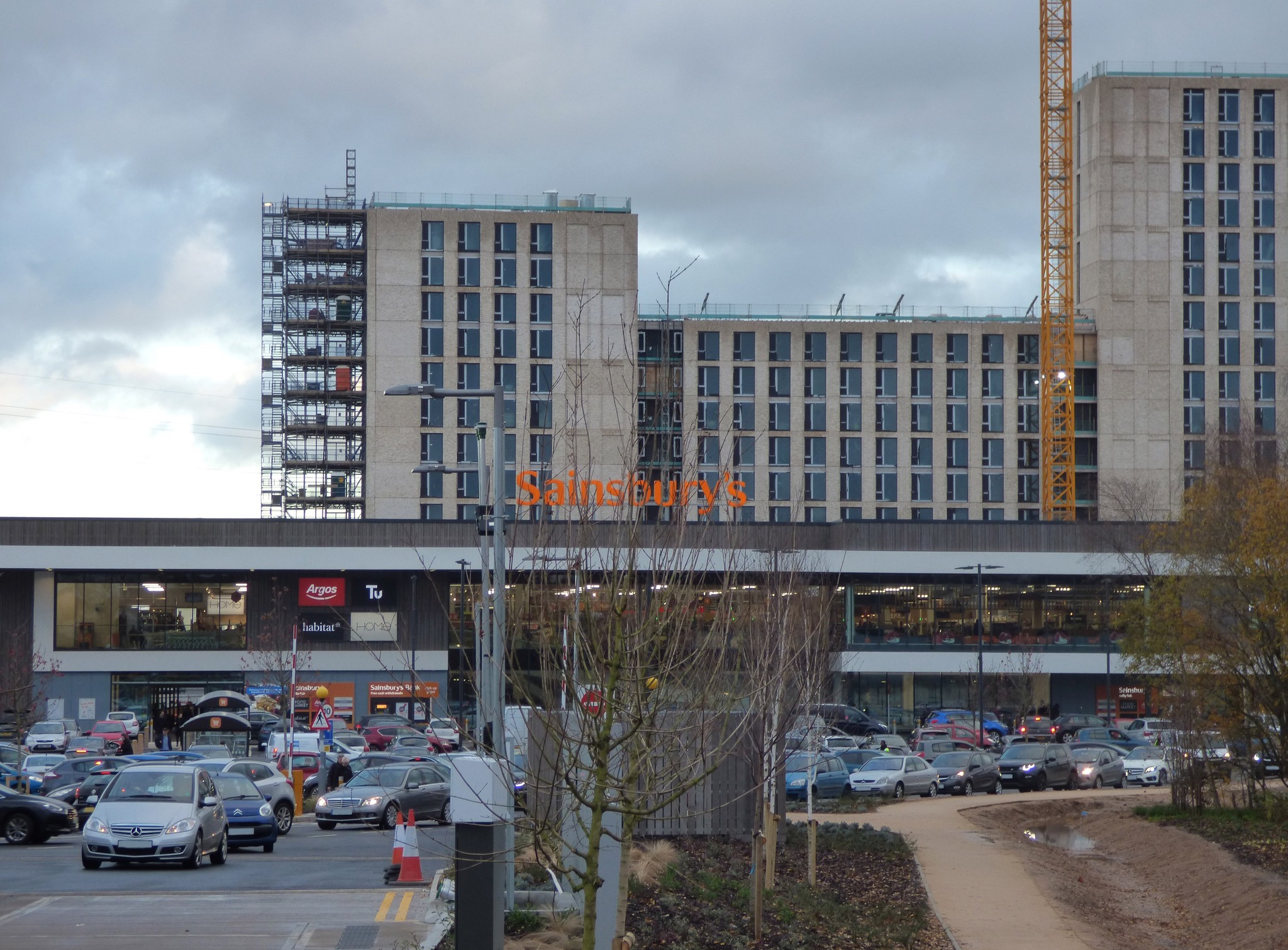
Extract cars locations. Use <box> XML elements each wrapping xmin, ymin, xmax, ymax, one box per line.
<box><xmin>785</xmin><ymin>704</ymin><xmax>1284</xmax><ymax>798</ymax></box>
<box><xmin>0</xmin><ymin>706</ymin><xmax>542</xmax><ymax>871</ymax></box>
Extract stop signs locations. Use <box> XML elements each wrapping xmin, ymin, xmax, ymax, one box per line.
<box><xmin>578</xmin><ymin>685</ymin><xmax>615</xmax><ymax>719</ymax></box>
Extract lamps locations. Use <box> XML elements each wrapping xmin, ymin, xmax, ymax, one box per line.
<box><xmin>206</xmin><ymin>587</ymin><xmax>215</xmax><ymax>599</ymax></box>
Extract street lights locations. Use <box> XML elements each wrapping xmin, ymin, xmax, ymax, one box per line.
<box><xmin>456</xmin><ymin>560</ymin><xmax>471</xmax><ymax>753</ymax></box>
<box><xmin>521</xmin><ymin>554</ymin><xmax>581</xmax><ymax>715</ymax></box>
<box><xmin>955</xmin><ymin>564</ymin><xmax>1004</xmax><ymax>749</ymax></box>
<box><xmin>317</xmin><ymin>687</ymin><xmax>329</xmax><ymax>800</ymax></box>
<box><xmin>754</xmin><ymin>548</ymin><xmax>801</xmax><ymax>626</ymax></box>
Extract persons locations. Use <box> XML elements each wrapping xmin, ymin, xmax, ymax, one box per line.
<box><xmin>879</xmin><ymin>741</ymin><xmax>888</xmax><ymax>751</ymax></box>
<box><xmin>117</xmin><ymin>610</ymin><xmax>187</xmax><ymax>650</ymax></box>
<box><xmin>326</xmin><ymin>754</ymin><xmax>353</xmax><ymax>792</ymax></box>
<box><xmin>119</xmin><ymin>733</ymin><xmax>134</xmax><ymax>756</ymax></box>
<box><xmin>303</xmin><ymin>758</ymin><xmax>312</xmax><ymax>767</ymax></box>
<box><xmin>973</xmin><ymin>619</ymin><xmax>984</xmax><ymax>635</ymax></box>
<box><xmin>152</xmin><ymin>705</ymin><xmax>194</xmax><ymax>752</ymax></box>
<box><xmin>1038</xmin><ymin>701</ymin><xmax>1049</xmax><ymax>716</ymax></box>
<box><xmin>922</xmin><ymin>704</ymin><xmax>929</xmax><ymax>713</ymax></box>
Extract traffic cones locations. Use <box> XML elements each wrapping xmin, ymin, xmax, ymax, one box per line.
<box><xmin>383</xmin><ymin>809</ymin><xmax>432</xmax><ymax>885</ymax></box>
